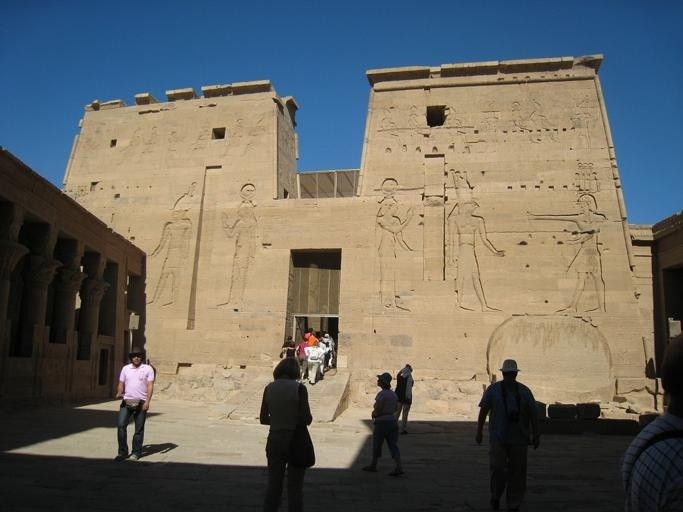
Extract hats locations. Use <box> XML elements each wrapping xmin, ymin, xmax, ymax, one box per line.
<box><xmin>500</xmin><ymin>360</ymin><xmax>520</xmax><ymax>372</ymax></box>
<box><xmin>377</xmin><ymin>373</ymin><xmax>392</xmax><ymax>382</ymax></box>
<box><xmin>129</xmin><ymin>346</ymin><xmax>144</xmax><ymax>357</ymax></box>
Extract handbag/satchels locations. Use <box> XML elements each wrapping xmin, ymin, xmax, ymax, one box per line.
<box><xmin>125</xmin><ymin>399</ymin><xmax>140</xmax><ymax>408</ymax></box>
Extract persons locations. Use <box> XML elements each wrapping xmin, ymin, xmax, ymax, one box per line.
<box><xmin>393</xmin><ymin>364</ymin><xmax>414</xmax><ymax>434</ymax></box>
<box><xmin>115</xmin><ymin>347</ymin><xmax>154</xmax><ymax>461</ymax></box>
<box><xmin>217</xmin><ymin>200</ymin><xmax>259</xmax><ymax>308</ymax></box>
<box><xmin>526</xmin><ymin>194</ymin><xmax>606</xmax><ymax>314</ymax></box>
<box><xmin>376</xmin><ymin>195</ymin><xmax>415</xmax><ymax>313</ymax></box>
<box><xmin>282</xmin><ymin>328</ymin><xmax>335</xmax><ymax>385</ymax></box>
<box><xmin>475</xmin><ymin>359</ymin><xmax>541</xmax><ymax>512</ymax></box>
<box><xmin>621</xmin><ymin>334</ymin><xmax>683</xmax><ymax>512</ymax></box>
<box><xmin>147</xmin><ymin>211</ymin><xmax>192</xmax><ymax>306</ymax></box>
<box><xmin>362</xmin><ymin>373</ymin><xmax>404</xmax><ymax>476</ymax></box>
<box><xmin>260</xmin><ymin>358</ymin><xmax>315</xmax><ymax>512</ymax></box>
<box><xmin>448</xmin><ymin>169</ymin><xmax>505</xmax><ymax>312</ymax></box>
<box><xmin>381</xmin><ymin>95</ymin><xmax>593</xmax><ymax>146</ymax></box>
